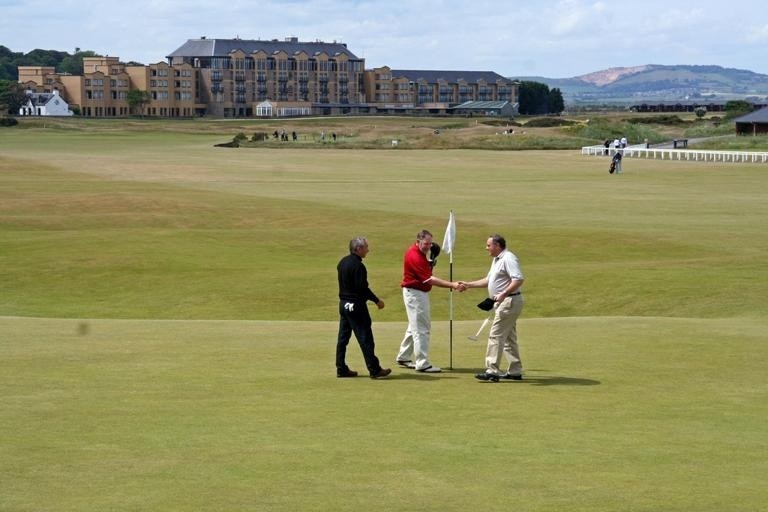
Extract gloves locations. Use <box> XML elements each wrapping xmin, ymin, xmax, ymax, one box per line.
<box><xmin>344</xmin><ymin>302</ymin><xmax>355</xmax><ymax>311</ymax></box>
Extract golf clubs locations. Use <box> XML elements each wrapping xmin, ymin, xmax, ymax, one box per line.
<box><xmin>468</xmin><ymin>302</ymin><xmax>499</xmax><ymax>341</ymax></box>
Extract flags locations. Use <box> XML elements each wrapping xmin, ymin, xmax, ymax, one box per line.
<box><xmin>437</xmin><ymin>213</ymin><xmax>464</xmax><ymax>262</ymax></box>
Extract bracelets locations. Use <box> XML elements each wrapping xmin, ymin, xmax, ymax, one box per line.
<box><xmin>503</xmin><ymin>290</ymin><xmax>509</xmax><ymax>295</ymax></box>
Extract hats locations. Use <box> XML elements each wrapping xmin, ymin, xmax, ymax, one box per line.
<box><xmin>477</xmin><ymin>298</ymin><xmax>497</xmax><ymax>311</ymax></box>
<box><xmin>430</xmin><ymin>242</ymin><xmax>440</xmax><ymax>260</ymax></box>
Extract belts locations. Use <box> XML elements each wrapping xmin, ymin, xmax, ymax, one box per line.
<box><xmin>507</xmin><ymin>292</ymin><xmax>520</xmax><ymax>297</ymax></box>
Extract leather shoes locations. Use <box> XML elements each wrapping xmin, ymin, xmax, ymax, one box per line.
<box><xmin>337</xmin><ymin>367</ymin><xmax>357</xmax><ymax>377</ymax></box>
<box><xmin>370</xmin><ymin>368</ymin><xmax>391</xmax><ymax>378</ymax></box>
<box><xmin>398</xmin><ymin>361</ymin><xmax>415</xmax><ymax>368</ymax></box>
<box><xmin>424</xmin><ymin>366</ymin><xmax>441</xmax><ymax>373</ymax></box>
<box><xmin>499</xmin><ymin>373</ymin><xmax>521</xmax><ymax>380</ymax></box>
<box><xmin>474</xmin><ymin>372</ymin><xmax>499</xmax><ymax>382</ymax></box>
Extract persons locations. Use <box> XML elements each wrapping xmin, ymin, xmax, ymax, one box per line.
<box><xmin>455</xmin><ymin>232</ymin><xmax>526</xmax><ymax>382</ymax></box>
<box><xmin>332</xmin><ymin>235</ymin><xmax>392</xmax><ymax>379</ymax></box>
<box><xmin>619</xmin><ymin>136</ymin><xmax>627</xmax><ymax>148</ymax></box>
<box><xmin>395</xmin><ymin>228</ymin><xmax>468</xmax><ymax>374</ymax></box>
<box><xmin>613</xmin><ymin>138</ymin><xmax>620</xmax><ymax>149</ymax></box>
<box><xmin>331</xmin><ymin>133</ymin><xmax>338</xmax><ymax>142</ymax></box>
<box><xmin>271</xmin><ymin>129</ymin><xmax>298</xmax><ymax>142</ymax></box>
<box><xmin>611</xmin><ymin>152</ymin><xmax>623</xmax><ymax>174</ymax></box>
<box><xmin>604</xmin><ymin>138</ymin><xmax>611</xmax><ymax>155</ymax></box>
<box><xmin>318</xmin><ymin>130</ymin><xmax>326</xmax><ymax>140</ymax></box>
<box><xmin>644</xmin><ymin>137</ymin><xmax>649</xmax><ymax>150</ymax></box>
